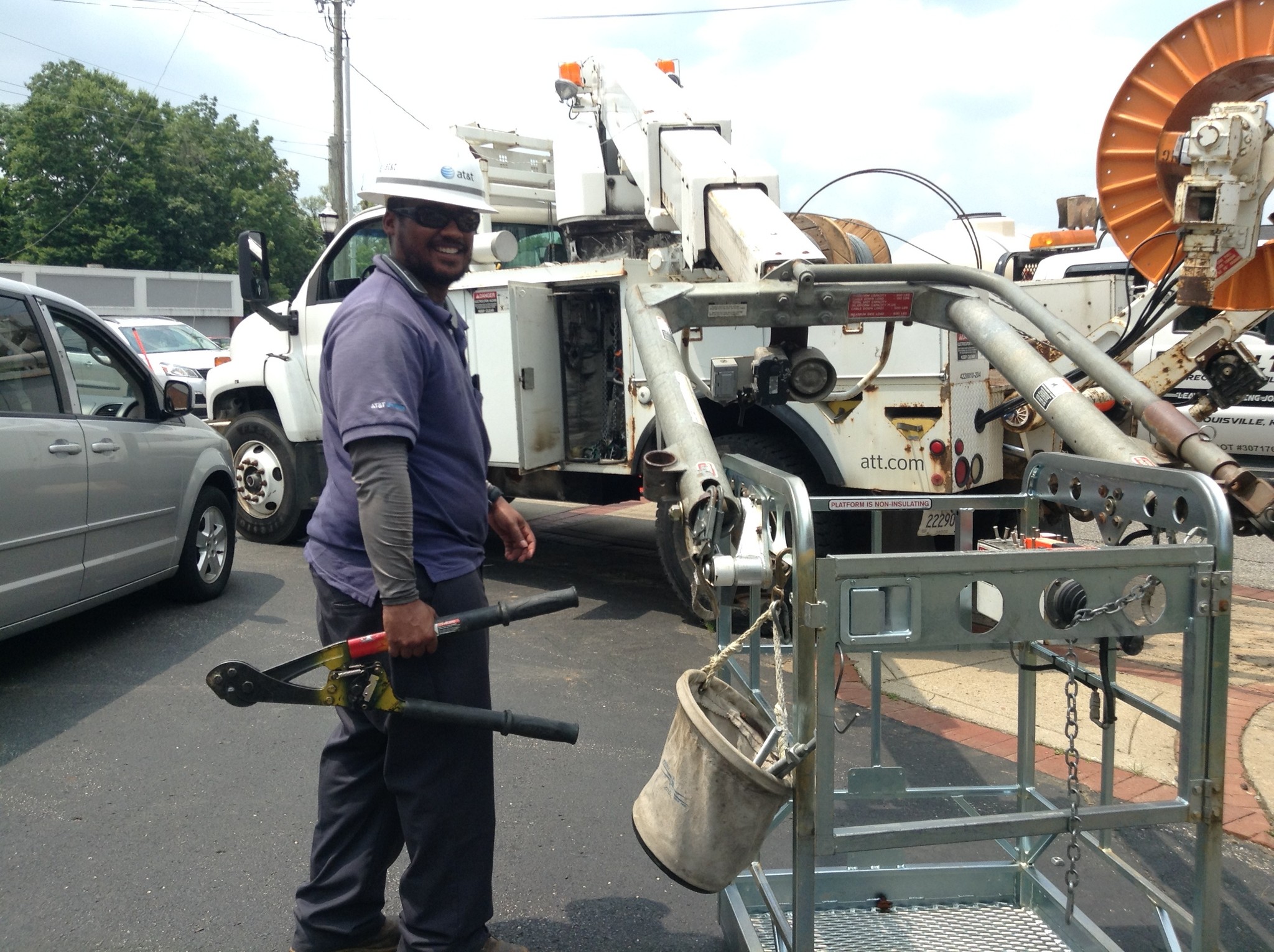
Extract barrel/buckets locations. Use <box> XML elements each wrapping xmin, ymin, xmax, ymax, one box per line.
<box><xmin>631</xmin><ymin>608</ymin><xmax>792</xmax><ymax>894</ymax></box>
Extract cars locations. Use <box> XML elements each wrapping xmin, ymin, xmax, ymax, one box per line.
<box><xmin>11</xmin><ymin>313</ymin><xmax>230</xmax><ymax>427</ymax></box>
<box><xmin>0</xmin><ymin>275</ymin><xmax>241</xmax><ymax>647</ymax></box>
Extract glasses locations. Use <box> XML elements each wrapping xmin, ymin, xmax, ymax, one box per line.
<box><xmin>392</xmin><ymin>204</ymin><xmax>481</xmax><ymax>233</ymax></box>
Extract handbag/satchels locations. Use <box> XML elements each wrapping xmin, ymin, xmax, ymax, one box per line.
<box><xmin>630</xmin><ymin>598</ymin><xmax>796</xmax><ymax>895</ymax></box>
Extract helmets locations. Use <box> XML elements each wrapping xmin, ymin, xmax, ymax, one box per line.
<box><xmin>357</xmin><ymin>128</ymin><xmax>502</xmax><ymax>215</ymax></box>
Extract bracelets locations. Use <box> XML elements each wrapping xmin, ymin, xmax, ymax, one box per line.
<box><xmin>487</xmin><ymin>486</ymin><xmax>503</xmax><ymax>514</ymax></box>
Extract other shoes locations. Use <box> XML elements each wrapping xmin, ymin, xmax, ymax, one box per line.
<box><xmin>288</xmin><ymin>914</ymin><xmax>403</xmax><ymax>952</ymax></box>
<box><xmin>477</xmin><ymin>936</ymin><xmax>530</xmax><ymax>952</ymax></box>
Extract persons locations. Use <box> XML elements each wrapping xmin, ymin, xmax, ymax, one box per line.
<box><xmin>290</xmin><ymin>129</ymin><xmax>536</xmax><ymax>952</ymax></box>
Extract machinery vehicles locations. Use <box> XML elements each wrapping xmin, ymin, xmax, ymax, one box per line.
<box><xmin>201</xmin><ymin>1</ymin><xmax>1274</xmax><ymax>639</ymax></box>
